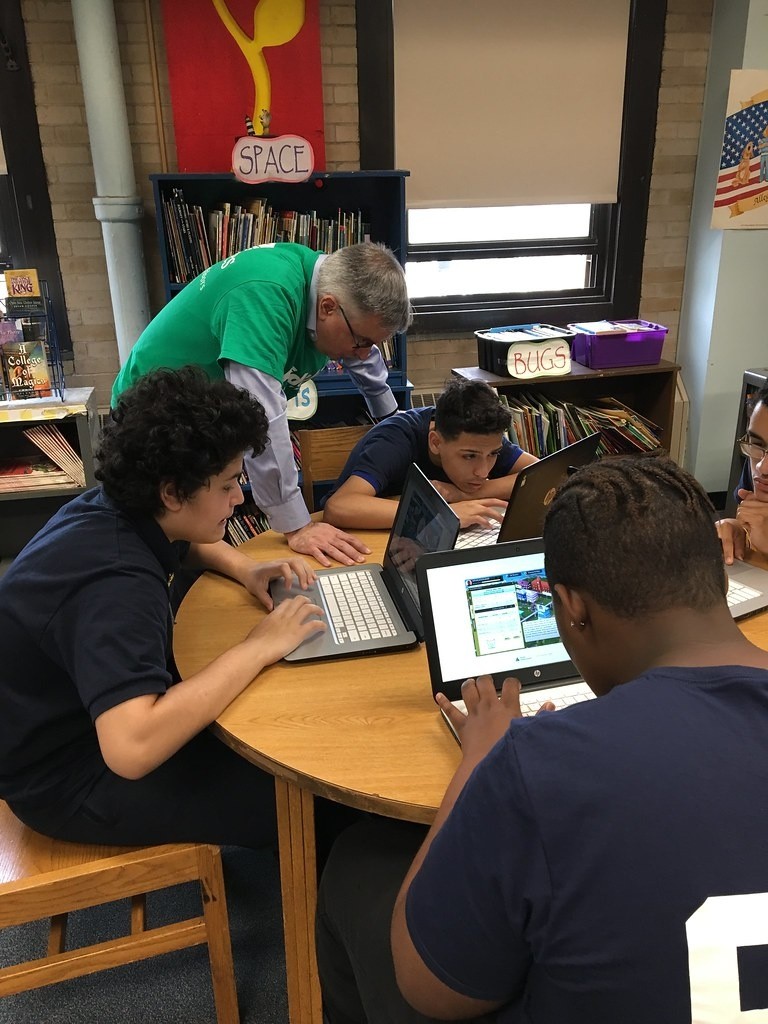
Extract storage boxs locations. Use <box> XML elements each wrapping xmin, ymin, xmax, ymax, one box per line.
<box><xmin>567</xmin><ymin>320</ymin><xmax>669</xmax><ymax>370</ymax></box>
<box><xmin>473</xmin><ymin>321</ymin><xmax>578</xmax><ymax>380</ymax></box>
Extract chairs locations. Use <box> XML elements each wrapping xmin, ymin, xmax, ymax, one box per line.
<box><xmin>297</xmin><ymin>425</ymin><xmax>376</xmax><ymax>514</ymax></box>
<box><xmin>0</xmin><ymin>799</ymin><xmax>240</xmax><ymax>1024</ymax></box>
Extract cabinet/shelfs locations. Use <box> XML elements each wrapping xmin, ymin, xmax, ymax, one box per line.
<box><xmin>0</xmin><ymin>386</ymin><xmax>102</xmax><ymax>582</ymax></box>
<box><xmin>148</xmin><ymin>167</ymin><xmax>415</xmax><ymax>515</ymax></box>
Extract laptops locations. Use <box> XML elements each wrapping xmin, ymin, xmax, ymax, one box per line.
<box><xmin>268</xmin><ymin>430</ymin><xmax>768</xmax><ymax>751</ymax></box>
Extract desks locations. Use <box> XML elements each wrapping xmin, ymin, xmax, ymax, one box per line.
<box><xmin>171</xmin><ymin>510</ymin><xmax>768</xmax><ymax>1024</ymax></box>
<box><xmin>451</xmin><ymin>358</ymin><xmax>683</xmax><ymax>462</ymax></box>
<box><xmin>723</xmin><ymin>367</ymin><xmax>768</xmax><ymax>518</ymax></box>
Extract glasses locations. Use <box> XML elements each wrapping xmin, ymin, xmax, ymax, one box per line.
<box><xmin>737</xmin><ymin>433</ymin><xmax>768</xmax><ymax>459</ymax></box>
<box><xmin>339</xmin><ymin>304</ymin><xmax>373</xmax><ymax>349</ymax></box>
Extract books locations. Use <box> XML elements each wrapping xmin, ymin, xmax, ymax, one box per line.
<box><xmin>226</xmin><ymin>511</ymin><xmax>271</xmax><ymax>549</ymax></box>
<box><xmin>497</xmin><ymin>390</ymin><xmax>664</xmax><ymax>458</ymax></box>
<box><xmin>0</xmin><ymin>424</ymin><xmax>85</xmax><ymax>490</ymax></box>
<box><xmin>163</xmin><ymin>189</ymin><xmax>398</xmax><ymax>375</ymax></box>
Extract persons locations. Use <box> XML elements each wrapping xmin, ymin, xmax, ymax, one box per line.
<box><xmin>325</xmin><ymin>376</ymin><xmax>538</xmax><ymax>529</ymax></box>
<box><xmin>105</xmin><ymin>236</ymin><xmax>413</xmax><ymax>566</ymax></box>
<box><xmin>0</xmin><ymin>362</ymin><xmax>328</xmax><ymax>852</ymax></box>
<box><xmin>712</xmin><ymin>375</ymin><xmax>768</xmax><ymax>567</ymax></box>
<box><xmin>388</xmin><ymin>443</ymin><xmax>768</xmax><ymax>1022</ymax></box>
